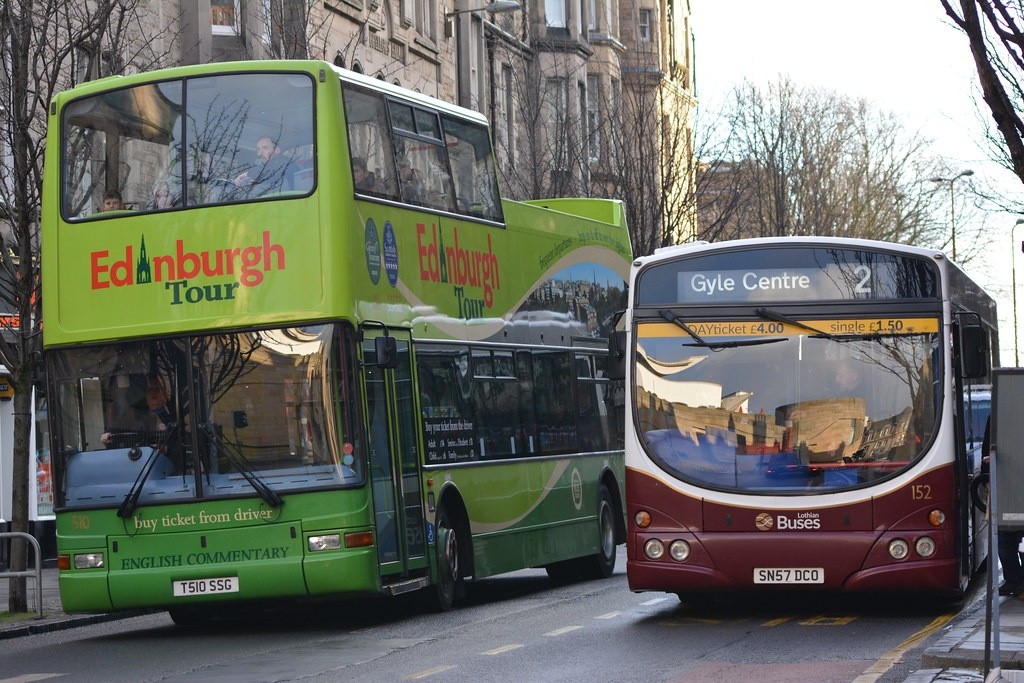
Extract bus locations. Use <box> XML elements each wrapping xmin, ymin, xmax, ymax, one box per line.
<box><xmin>605</xmin><ymin>233</ymin><xmax>1000</xmax><ymax>614</ymax></box>
<box><xmin>22</xmin><ymin>59</ymin><xmax>640</xmax><ymax>629</ymax></box>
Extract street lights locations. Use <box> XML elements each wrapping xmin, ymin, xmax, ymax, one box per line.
<box><xmin>929</xmin><ymin>169</ymin><xmax>974</xmax><ymax>264</ymax></box>
<box><xmin>1010</xmin><ymin>218</ymin><xmax>1024</xmax><ymax>371</ymax></box>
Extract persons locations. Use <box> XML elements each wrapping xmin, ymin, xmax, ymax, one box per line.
<box><xmin>352</xmin><ymin>157</ymin><xmax>386</xmax><ymax>200</ymax></box>
<box><xmin>396</xmin><ymin>153</ymin><xmax>446</xmax><ymax>208</ymax></box>
<box><xmin>828</xmin><ymin>359</ymin><xmax>872</xmax><ymax>426</ymax></box>
<box><xmin>980</xmin><ymin>415</ymin><xmax>1024</xmax><ymax>595</ymax></box>
<box><xmin>152</xmin><ymin>180</ymin><xmax>180</xmax><ymax>209</ymax></box>
<box><xmin>236</xmin><ymin>135</ymin><xmax>301</xmax><ymax>198</ymax></box>
<box><xmin>101</xmin><ymin>188</ymin><xmax>126</xmax><ymax>212</ymax></box>
<box><xmin>100</xmin><ymin>374</ymin><xmax>183</xmax><ymax>477</ymax></box>
